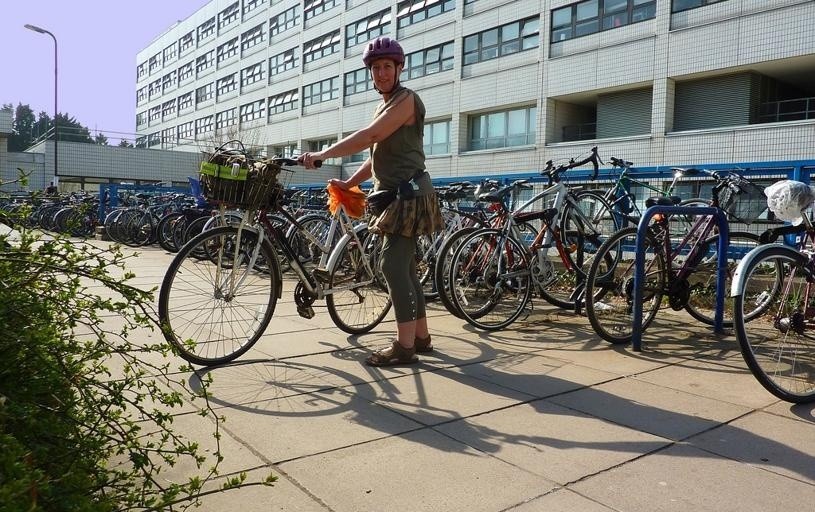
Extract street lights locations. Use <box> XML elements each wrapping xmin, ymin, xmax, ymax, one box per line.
<box><xmin>24</xmin><ymin>23</ymin><xmax>59</xmax><ymax>191</ymax></box>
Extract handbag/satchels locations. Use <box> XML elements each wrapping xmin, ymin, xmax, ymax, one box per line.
<box><xmin>367</xmin><ymin>189</ymin><xmax>396</xmax><ymax>218</ymax></box>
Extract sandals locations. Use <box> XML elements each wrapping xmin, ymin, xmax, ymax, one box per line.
<box><xmin>365</xmin><ymin>340</ymin><xmax>419</xmax><ymax>367</ymax></box>
<box><xmin>414</xmin><ymin>334</ymin><xmax>433</xmax><ymax>352</ymax></box>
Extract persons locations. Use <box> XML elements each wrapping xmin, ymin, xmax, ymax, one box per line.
<box><xmin>294</xmin><ymin>38</ymin><xmax>447</xmax><ymax>368</ymax></box>
<box><xmin>44</xmin><ymin>181</ymin><xmax>58</xmax><ymax>195</ymax></box>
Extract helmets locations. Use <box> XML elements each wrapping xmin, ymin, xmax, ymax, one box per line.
<box><xmin>362</xmin><ymin>36</ymin><xmax>405</xmax><ymax>69</ymax></box>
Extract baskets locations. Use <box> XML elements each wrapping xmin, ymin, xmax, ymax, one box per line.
<box><xmin>196</xmin><ymin>150</ymin><xmax>296</xmax><ymax>215</ymax></box>
